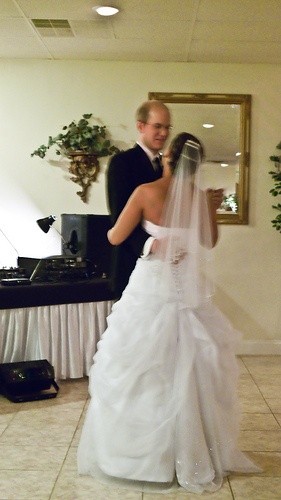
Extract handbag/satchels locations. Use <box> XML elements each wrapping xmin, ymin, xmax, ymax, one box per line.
<box><xmin>0</xmin><ymin>359</ymin><xmax>60</xmax><ymax>403</ymax></box>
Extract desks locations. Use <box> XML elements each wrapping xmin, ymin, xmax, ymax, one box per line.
<box><xmin>0</xmin><ymin>270</ymin><xmax>136</xmax><ymax>385</ymax></box>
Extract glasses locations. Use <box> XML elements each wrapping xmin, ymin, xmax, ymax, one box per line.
<box><xmin>138</xmin><ymin>120</ymin><xmax>174</xmax><ymax>133</ymax></box>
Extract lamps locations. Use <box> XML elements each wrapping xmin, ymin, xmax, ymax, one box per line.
<box><xmin>36</xmin><ymin>215</ymin><xmax>75</xmax><ymax>251</ymax></box>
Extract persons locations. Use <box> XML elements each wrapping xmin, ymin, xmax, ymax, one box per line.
<box><xmin>76</xmin><ymin>132</ymin><xmax>266</xmax><ymax>494</ymax></box>
<box><xmin>107</xmin><ymin>99</ymin><xmax>224</xmax><ymax>304</ymax></box>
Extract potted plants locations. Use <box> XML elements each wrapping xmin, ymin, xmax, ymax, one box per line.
<box><xmin>31</xmin><ymin>114</ymin><xmax>126</xmax><ymax>202</ymax></box>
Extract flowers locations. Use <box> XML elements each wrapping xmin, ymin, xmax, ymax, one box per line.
<box><xmin>219</xmin><ymin>194</ymin><xmax>240</xmax><ymax>214</ymax></box>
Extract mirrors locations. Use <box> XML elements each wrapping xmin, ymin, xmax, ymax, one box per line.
<box><xmin>148</xmin><ymin>92</ymin><xmax>252</xmax><ymax>225</ymax></box>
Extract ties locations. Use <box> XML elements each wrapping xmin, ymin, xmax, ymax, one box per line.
<box><xmin>152</xmin><ymin>156</ymin><xmax>163</xmax><ymax>179</ymax></box>
